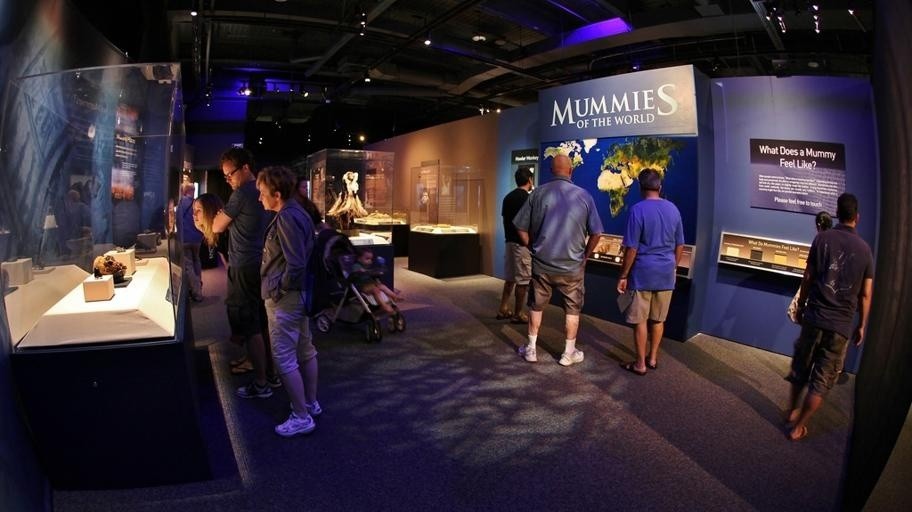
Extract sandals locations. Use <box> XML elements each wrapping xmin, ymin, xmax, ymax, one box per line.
<box><xmin>496</xmin><ymin>309</ymin><xmax>515</xmax><ymax>319</ymax></box>
<box><xmin>511</xmin><ymin>312</ymin><xmax>529</xmax><ymax>324</ymax></box>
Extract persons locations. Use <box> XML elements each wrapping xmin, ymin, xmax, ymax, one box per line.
<box><xmin>256</xmin><ymin>168</ymin><xmax>323</xmax><ymax>437</ymax></box>
<box><xmin>497</xmin><ymin>166</ymin><xmax>535</xmax><ymax>324</ymax></box>
<box><xmin>174</xmin><ymin>182</ymin><xmax>206</xmax><ymax>304</ymax></box>
<box><xmin>517</xmin><ymin>154</ymin><xmax>602</xmax><ymax>367</ymax></box>
<box><xmin>784</xmin><ymin>193</ymin><xmax>873</xmax><ymax>442</ymax></box>
<box><xmin>211</xmin><ymin>146</ymin><xmax>283</xmax><ymax>399</ymax></box>
<box><xmin>616</xmin><ymin>168</ymin><xmax>684</xmax><ymax>376</ymax></box>
<box><xmin>352</xmin><ymin>247</ymin><xmax>405</xmax><ymax>315</ymax></box>
<box><xmin>786</xmin><ymin>211</ymin><xmax>848</xmax><ymax>386</ymax></box>
<box><xmin>294</xmin><ymin>175</ymin><xmax>322</xmax><ymax>228</ymax></box>
<box><xmin>191</xmin><ymin>193</ymin><xmax>255</xmax><ymax>375</ymax></box>
<box><xmin>330</xmin><ymin>172</ymin><xmax>367</xmax><ymax>216</ymax></box>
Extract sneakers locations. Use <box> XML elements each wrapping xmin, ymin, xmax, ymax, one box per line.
<box><xmin>275</xmin><ymin>412</ymin><xmax>315</xmax><ymax>436</ymax></box>
<box><xmin>234</xmin><ymin>383</ymin><xmax>271</xmax><ymax>399</ymax></box>
<box><xmin>289</xmin><ymin>401</ymin><xmax>322</xmax><ymax>415</ymax></box>
<box><xmin>266</xmin><ymin>374</ymin><xmax>281</xmax><ymax>386</ymax></box>
<box><xmin>229</xmin><ymin>356</ymin><xmax>255</xmax><ymax>374</ymax></box>
<box><xmin>517</xmin><ymin>344</ymin><xmax>536</xmax><ymax>361</ymax></box>
<box><xmin>558</xmin><ymin>350</ymin><xmax>586</xmax><ymax>366</ymax></box>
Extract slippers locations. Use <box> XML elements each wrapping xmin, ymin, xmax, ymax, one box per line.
<box><xmin>785</xmin><ymin>408</ymin><xmax>795</xmax><ymax>429</ymax></box>
<box><xmin>645</xmin><ymin>357</ymin><xmax>657</xmax><ymax>370</ymax></box>
<box><xmin>789</xmin><ymin>425</ymin><xmax>808</xmax><ymax>441</ymax></box>
<box><xmin>619</xmin><ymin>360</ymin><xmax>647</xmax><ymax>376</ymax></box>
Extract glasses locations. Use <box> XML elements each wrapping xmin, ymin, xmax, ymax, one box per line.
<box><xmin>223</xmin><ymin>168</ymin><xmax>244</xmax><ymax>178</ymax></box>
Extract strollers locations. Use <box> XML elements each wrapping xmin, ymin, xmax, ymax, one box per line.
<box><xmin>313</xmin><ymin>229</ymin><xmax>406</xmax><ymax>343</ymax></box>
